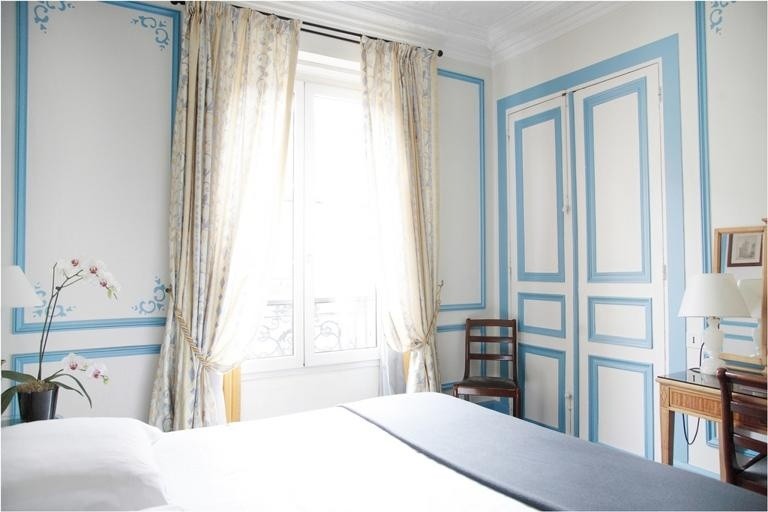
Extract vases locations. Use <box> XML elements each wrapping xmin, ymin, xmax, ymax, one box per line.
<box><xmin>18</xmin><ymin>383</ymin><xmax>58</xmax><ymax>422</ymax></box>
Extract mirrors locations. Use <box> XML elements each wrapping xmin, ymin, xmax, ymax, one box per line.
<box><xmin>712</xmin><ymin>225</ymin><xmax>768</xmax><ymax>365</ymax></box>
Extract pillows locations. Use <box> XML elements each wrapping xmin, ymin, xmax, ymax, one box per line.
<box><xmin>0</xmin><ymin>417</ymin><xmax>168</xmax><ymax>512</ymax></box>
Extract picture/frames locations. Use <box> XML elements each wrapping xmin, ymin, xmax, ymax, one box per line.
<box><xmin>726</xmin><ymin>234</ymin><xmax>762</xmax><ymax>267</ymax></box>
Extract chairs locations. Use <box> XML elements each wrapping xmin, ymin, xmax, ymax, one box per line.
<box><xmin>715</xmin><ymin>368</ymin><xmax>768</xmax><ymax>498</ymax></box>
<box><xmin>451</xmin><ymin>318</ymin><xmax>520</xmax><ymax>420</ymax></box>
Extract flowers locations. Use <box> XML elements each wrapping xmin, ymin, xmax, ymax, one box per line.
<box><xmin>1</xmin><ymin>255</ymin><xmax>122</xmax><ymax>415</ymax></box>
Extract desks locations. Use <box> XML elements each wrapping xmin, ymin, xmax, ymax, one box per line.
<box><xmin>655</xmin><ymin>366</ymin><xmax>768</xmax><ymax>467</ymax></box>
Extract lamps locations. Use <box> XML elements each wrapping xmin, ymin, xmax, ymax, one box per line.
<box><xmin>678</xmin><ymin>272</ymin><xmax>752</xmax><ymax>376</ymax></box>
<box><xmin>1</xmin><ymin>265</ymin><xmax>43</xmax><ymax>309</ymax></box>
<box><xmin>735</xmin><ymin>278</ymin><xmax>763</xmax><ymax>358</ymax></box>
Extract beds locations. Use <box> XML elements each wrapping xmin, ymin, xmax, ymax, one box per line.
<box><xmin>165</xmin><ymin>389</ymin><xmax>768</xmax><ymax>512</ymax></box>
